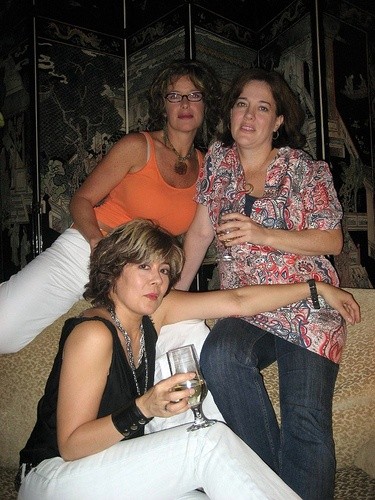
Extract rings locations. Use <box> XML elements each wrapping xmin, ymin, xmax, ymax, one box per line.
<box><xmin>165</xmin><ymin>405</ymin><xmax>167</xmax><ymax>410</ymax></box>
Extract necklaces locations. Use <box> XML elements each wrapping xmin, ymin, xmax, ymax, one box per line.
<box><xmin>109</xmin><ymin>309</ymin><xmax>149</xmax><ymax>396</ymax></box>
<box><xmin>163</xmin><ymin>127</ymin><xmax>194</xmax><ymax>175</ymax></box>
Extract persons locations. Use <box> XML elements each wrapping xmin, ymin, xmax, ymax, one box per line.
<box><xmin>18</xmin><ymin>218</ymin><xmax>360</xmax><ymax>500</ymax></box>
<box><xmin>0</xmin><ymin>60</ymin><xmax>224</xmax><ymax>354</ymax></box>
<box><xmin>171</xmin><ymin>70</ymin><xmax>347</xmax><ymax>500</ymax></box>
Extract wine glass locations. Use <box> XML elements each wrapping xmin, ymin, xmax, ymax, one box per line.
<box><xmin>215</xmin><ymin>211</ymin><xmax>236</xmax><ymax>263</ymax></box>
<box><xmin>166</xmin><ymin>342</ymin><xmax>217</xmax><ymax>432</ymax></box>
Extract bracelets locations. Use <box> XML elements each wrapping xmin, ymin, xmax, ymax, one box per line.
<box><xmin>111</xmin><ymin>399</ymin><xmax>154</xmax><ymax>437</ymax></box>
<box><xmin>307</xmin><ymin>279</ymin><xmax>320</xmax><ymax>309</ymax></box>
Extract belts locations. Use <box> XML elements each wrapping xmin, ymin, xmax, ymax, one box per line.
<box><xmin>13</xmin><ymin>462</ymin><xmax>33</xmax><ymax>492</ymax></box>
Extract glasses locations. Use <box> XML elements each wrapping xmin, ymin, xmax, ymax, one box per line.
<box><xmin>164</xmin><ymin>92</ymin><xmax>203</xmax><ymax>103</ymax></box>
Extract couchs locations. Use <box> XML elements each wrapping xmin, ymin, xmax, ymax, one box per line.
<box><xmin>0</xmin><ymin>286</ymin><xmax>375</xmax><ymax>500</ymax></box>
